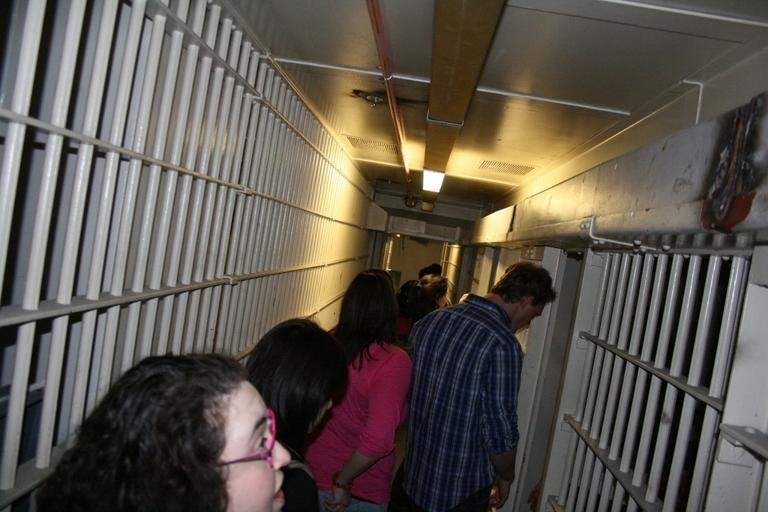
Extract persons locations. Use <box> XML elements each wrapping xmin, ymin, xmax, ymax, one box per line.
<box><xmin>308</xmin><ymin>266</ymin><xmax>413</xmax><ymax>512</ymax></box>
<box><xmin>246</xmin><ymin>318</ymin><xmax>348</xmax><ymax>512</ymax></box>
<box><xmin>397</xmin><ymin>264</ymin><xmax>448</xmax><ymax>341</ymax></box>
<box><xmin>36</xmin><ymin>351</ymin><xmax>291</xmax><ymax>512</ymax></box>
<box><xmin>389</xmin><ymin>261</ymin><xmax>556</xmax><ymax>512</ymax></box>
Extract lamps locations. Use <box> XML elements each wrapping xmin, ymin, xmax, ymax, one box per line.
<box><xmin>421</xmin><ymin>168</ymin><xmax>446</xmax><ymax>193</ymax></box>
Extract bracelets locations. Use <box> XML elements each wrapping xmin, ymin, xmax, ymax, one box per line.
<box><xmin>334</xmin><ymin>478</ymin><xmax>353</xmax><ymax>489</ymax></box>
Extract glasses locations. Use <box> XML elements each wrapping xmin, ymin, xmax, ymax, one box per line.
<box><xmin>215</xmin><ymin>408</ymin><xmax>277</xmax><ymax>469</ymax></box>
